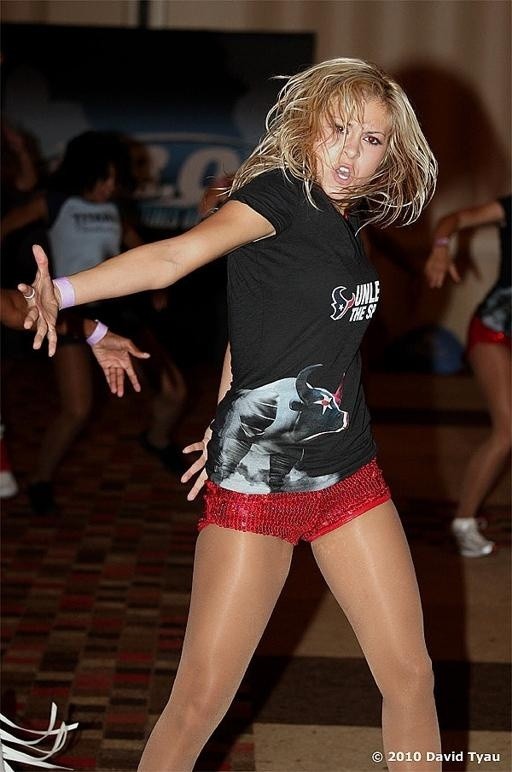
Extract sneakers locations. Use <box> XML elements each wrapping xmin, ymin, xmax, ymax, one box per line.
<box><xmin>138</xmin><ymin>429</ymin><xmax>186</xmax><ymax>472</ymax></box>
<box><xmin>17</xmin><ymin>480</ymin><xmax>60</xmax><ymax>514</ymax></box>
<box><xmin>449</xmin><ymin>515</ymin><xmax>496</xmax><ymax>558</ymax></box>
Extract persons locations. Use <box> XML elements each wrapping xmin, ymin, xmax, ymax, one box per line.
<box><xmin>0</xmin><ymin>116</ymin><xmax>511</xmax><ymax>561</ymax></box>
<box><xmin>17</xmin><ymin>52</ymin><xmax>449</xmax><ymax>772</ymax></box>
